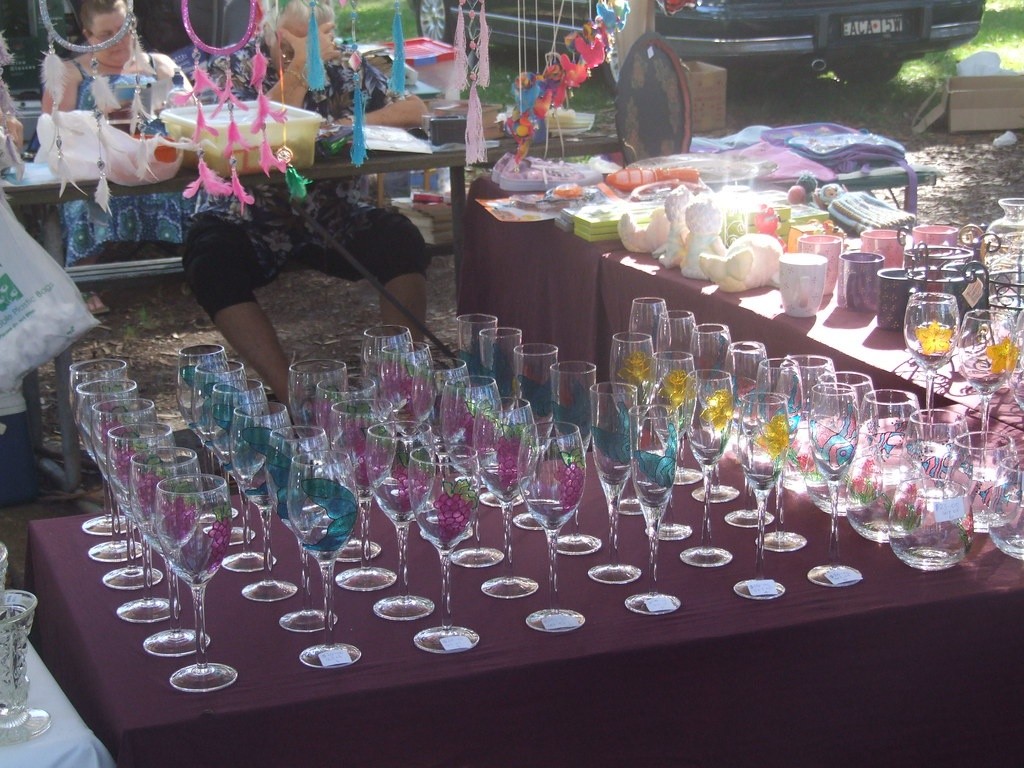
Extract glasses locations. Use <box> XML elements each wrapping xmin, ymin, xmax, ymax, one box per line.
<box><xmin>89</xmin><ymin>14</ymin><xmax>137</xmax><ymax>45</ymax></box>
<box><xmin>274</xmin><ymin>31</ymin><xmax>294</xmax><ymax>66</ymax></box>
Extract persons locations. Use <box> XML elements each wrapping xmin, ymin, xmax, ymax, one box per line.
<box><xmin>43</xmin><ymin>0</ymin><xmax>202</xmax><ymax>314</ymax></box>
<box><xmin>186</xmin><ymin>0</ymin><xmax>427</xmax><ymax>422</ymax></box>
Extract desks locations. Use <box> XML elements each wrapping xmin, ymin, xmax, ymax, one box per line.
<box><xmin>458</xmin><ymin>172</ymin><xmax>627</xmax><ymax>395</ymax></box>
<box><xmin>600</xmin><ymin>229</ymin><xmax>1024</xmax><ymax>441</ymax></box>
<box><xmin>23</xmin><ymin>439</ymin><xmax>1024</xmax><ymax>768</ymax></box>
<box><xmin>707</xmin><ymin>167</ymin><xmax>938</xmax><ymax>211</ymax></box>
<box><xmin>0</xmin><ymin>112</ymin><xmax>621</xmax><ymax>491</ymax></box>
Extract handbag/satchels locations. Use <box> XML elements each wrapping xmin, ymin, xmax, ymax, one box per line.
<box><xmin>0</xmin><ymin>187</ymin><xmax>103</xmax><ymax>396</ymax></box>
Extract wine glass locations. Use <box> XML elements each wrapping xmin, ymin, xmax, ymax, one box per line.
<box><xmin>69</xmin><ymin>297</ymin><xmax>861</xmax><ymax>696</ymax></box>
<box><xmin>903</xmin><ymin>292</ymin><xmax>1024</xmax><ymax>481</ymax></box>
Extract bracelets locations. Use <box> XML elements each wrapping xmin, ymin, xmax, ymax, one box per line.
<box><xmin>283</xmin><ymin>69</ymin><xmax>307</xmax><ymax>89</ymax></box>
<box><xmin>347</xmin><ymin>116</ymin><xmax>353</xmax><ymax>120</ymax></box>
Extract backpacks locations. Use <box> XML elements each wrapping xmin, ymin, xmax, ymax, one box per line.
<box><xmin>761</xmin><ymin>123</ymin><xmax>907</xmax><ymax>172</ymax></box>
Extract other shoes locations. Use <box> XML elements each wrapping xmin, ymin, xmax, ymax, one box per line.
<box><xmin>81</xmin><ymin>289</ymin><xmax>110</xmax><ymax>315</ymax></box>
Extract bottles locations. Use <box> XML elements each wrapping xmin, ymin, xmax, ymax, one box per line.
<box><xmin>980</xmin><ymin>197</ymin><xmax>1024</xmax><ymax>265</ymax></box>
<box><xmin>316</xmin><ymin>125</ymin><xmax>353</xmax><ymax>160</ymax></box>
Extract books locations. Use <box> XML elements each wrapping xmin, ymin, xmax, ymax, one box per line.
<box><xmin>115</xmin><ymin>77</ymin><xmax>172</xmax><ymax>117</ymax></box>
<box><xmin>390</xmin><ymin>192</ymin><xmax>453</xmax><ymax>244</ymax></box>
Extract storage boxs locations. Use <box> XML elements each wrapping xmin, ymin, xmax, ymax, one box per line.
<box><xmin>943</xmin><ymin>74</ymin><xmax>1024</xmax><ymax>134</ymax></box>
<box><xmin>655</xmin><ymin>58</ymin><xmax>727</xmax><ymax>134</ymax></box>
<box><xmin>157</xmin><ymin>101</ymin><xmax>322</xmax><ymax>178</ymax></box>
<box><xmin>386</xmin><ymin>36</ymin><xmax>462</xmax><ymax>102</ymax></box>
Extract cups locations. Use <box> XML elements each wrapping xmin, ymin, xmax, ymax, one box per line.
<box><xmin>0</xmin><ymin>590</ymin><xmax>52</xmax><ymax>747</ymax></box>
<box><xmin>875</xmin><ymin>268</ymin><xmax>911</xmax><ymax>331</ymax></box>
<box><xmin>838</xmin><ymin>252</ymin><xmax>885</xmax><ymax>313</ymax></box>
<box><xmin>859</xmin><ymin>229</ymin><xmax>906</xmax><ymax>270</ymax></box>
<box><xmin>777</xmin><ymin>253</ymin><xmax>828</xmax><ymax>317</ymax></box>
<box><xmin>798</xmin><ymin>235</ymin><xmax>842</xmax><ymax>298</ymax></box>
<box><xmin>904</xmin><ymin>248</ymin><xmax>974</xmax><ymax>328</ymax></box>
<box><xmin>774</xmin><ymin>355</ymin><xmax>1024</xmax><ymax>571</ymax></box>
<box><xmin>911</xmin><ymin>225</ymin><xmax>959</xmax><ymax>248</ymax></box>
<box><xmin>0</xmin><ymin>542</ymin><xmax>9</xmax><ymax>590</ymax></box>
